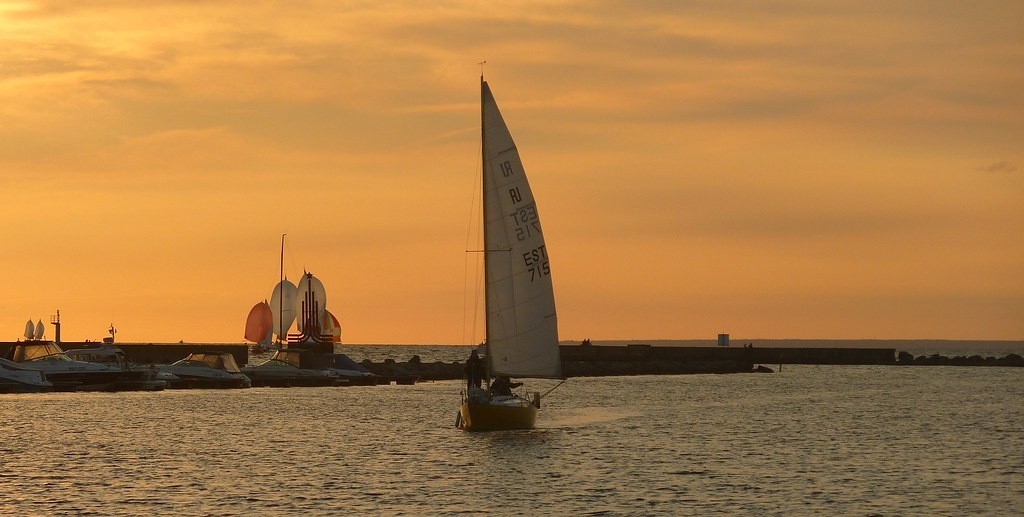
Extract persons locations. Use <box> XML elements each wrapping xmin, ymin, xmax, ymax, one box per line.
<box><xmin>583</xmin><ymin>338</ymin><xmax>590</xmax><ymax>345</ymax></box>
<box><xmin>464</xmin><ymin>350</ymin><xmax>523</xmax><ymax>396</ymax></box>
<box><xmin>744</xmin><ymin>343</ymin><xmax>753</xmax><ymax>351</ymax></box>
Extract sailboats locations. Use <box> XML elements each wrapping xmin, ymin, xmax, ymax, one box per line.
<box><xmin>455</xmin><ymin>63</ymin><xmax>570</xmax><ymax>431</ymax></box>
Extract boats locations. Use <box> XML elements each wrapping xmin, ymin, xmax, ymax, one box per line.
<box><xmin>0</xmin><ymin>235</ymin><xmax>383</xmax><ymax>391</ymax></box>
